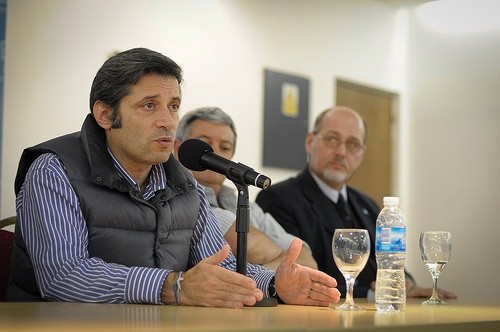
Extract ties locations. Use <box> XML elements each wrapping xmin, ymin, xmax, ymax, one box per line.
<box><xmin>335</xmin><ymin>193</ymin><xmax>359</xmax><ymax>228</ymax></box>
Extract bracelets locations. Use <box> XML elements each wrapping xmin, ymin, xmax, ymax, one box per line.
<box><xmin>268</xmin><ymin>276</ymin><xmax>286</xmax><ymax>304</ymax></box>
<box><xmin>172</xmin><ymin>271</ymin><xmax>183</xmax><ymax>305</ymax></box>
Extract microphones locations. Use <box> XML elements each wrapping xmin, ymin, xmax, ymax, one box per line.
<box><xmin>178</xmin><ymin>139</ymin><xmax>272</xmax><ymax>190</ymax></box>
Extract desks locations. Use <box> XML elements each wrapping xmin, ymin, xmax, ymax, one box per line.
<box><xmin>0</xmin><ymin>299</ymin><xmax>500</xmax><ymax>332</ymax></box>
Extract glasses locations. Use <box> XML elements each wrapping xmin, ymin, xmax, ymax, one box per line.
<box><xmin>315</xmin><ymin>133</ymin><xmax>367</xmax><ymax>155</ymax></box>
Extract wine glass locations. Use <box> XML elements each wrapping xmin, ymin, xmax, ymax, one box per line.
<box><xmin>419</xmin><ymin>230</ymin><xmax>451</xmax><ymax>305</ymax></box>
<box><xmin>330</xmin><ymin>227</ymin><xmax>371</xmax><ymax>312</ymax></box>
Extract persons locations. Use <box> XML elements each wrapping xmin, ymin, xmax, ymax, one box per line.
<box><xmin>14</xmin><ymin>47</ymin><xmax>341</xmax><ymax>308</ymax></box>
<box><xmin>173</xmin><ymin>105</ymin><xmax>318</xmax><ymax>271</ymax></box>
<box><xmin>255</xmin><ymin>106</ymin><xmax>458</xmax><ymax>299</ymax></box>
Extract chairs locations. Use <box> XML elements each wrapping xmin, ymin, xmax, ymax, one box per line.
<box><xmin>0</xmin><ymin>216</ymin><xmax>17</xmax><ymax>301</ymax></box>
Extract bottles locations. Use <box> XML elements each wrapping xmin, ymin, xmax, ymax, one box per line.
<box><xmin>375</xmin><ymin>197</ymin><xmax>407</xmax><ymax>310</ymax></box>
